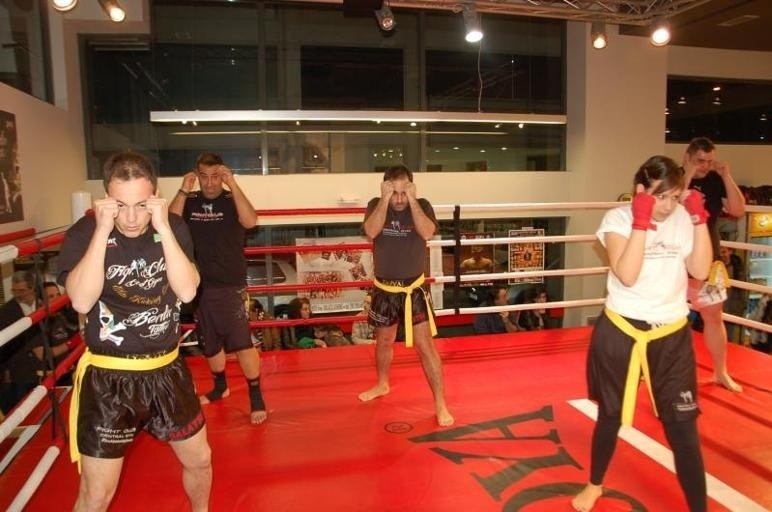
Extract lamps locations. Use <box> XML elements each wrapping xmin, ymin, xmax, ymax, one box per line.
<box><xmin>374</xmin><ymin>4</ymin><xmax>397</xmax><ymax>32</ymax></box>
<box><xmin>50</xmin><ymin>0</ymin><xmax>79</xmax><ymax>13</ymax></box>
<box><xmin>649</xmin><ymin>18</ymin><xmax>674</xmax><ymax>47</ymax></box>
<box><xmin>96</xmin><ymin>0</ymin><xmax>125</xmax><ymax>23</ymax></box>
<box><xmin>463</xmin><ymin>2</ymin><xmax>484</xmax><ymax>44</ymax></box>
<box><xmin>591</xmin><ymin>21</ymin><xmax>607</xmax><ymax>50</ymax></box>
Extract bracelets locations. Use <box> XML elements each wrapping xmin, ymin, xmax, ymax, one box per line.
<box><xmin>178</xmin><ymin>189</ymin><xmax>188</xmax><ymax>196</ymax></box>
<box><xmin>502</xmin><ymin>319</ymin><xmax>509</xmax><ymax>323</ymax></box>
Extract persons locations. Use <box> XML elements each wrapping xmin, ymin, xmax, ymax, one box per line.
<box><xmin>676</xmin><ymin>137</ymin><xmax>742</xmax><ymax>392</ymax></box>
<box><xmin>472</xmin><ymin>286</ymin><xmax>517</xmax><ymax>334</ymax></box>
<box><xmin>686</xmin><ymin>243</ymin><xmax>772</xmax><ymax>356</ymax></box>
<box><xmin>517</xmin><ymin>288</ymin><xmax>550</xmax><ymax>329</ymax></box>
<box><xmin>356</xmin><ymin>162</ymin><xmax>456</xmax><ymax>428</ymax></box>
<box><xmin>245</xmin><ymin>288</ymin><xmax>379</xmax><ymax>352</ymax></box>
<box><xmin>304</xmin><ymin>250</ymin><xmax>369</xmax><ymax>284</ymax></box>
<box><xmin>169</xmin><ymin>153</ymin><xmax>270</xmax><ymax>425</ymax></box>
<box><xmin>569</xmin><ymin>155</ymin><xmax>715</xmax><ymax>511</ymax></box>
<box><xmin>55</xmin><ymin>149</ymin><xmax>215</xmax><ymax>512</ymax></box>
<box><xmin>0</xmin><ymin>270</ymin><xmax>89</xmax><ymax>423</ymax></box>
<box><xmin>459</xmin><ymin>244</ymin><xmax>493</xmax><ymax>273</ymax></box>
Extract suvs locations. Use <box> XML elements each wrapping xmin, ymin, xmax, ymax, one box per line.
<box><xmin>244</xmin><ymin>258</ymin><xmax>297</xmax><ymax>321</ymax></box>
